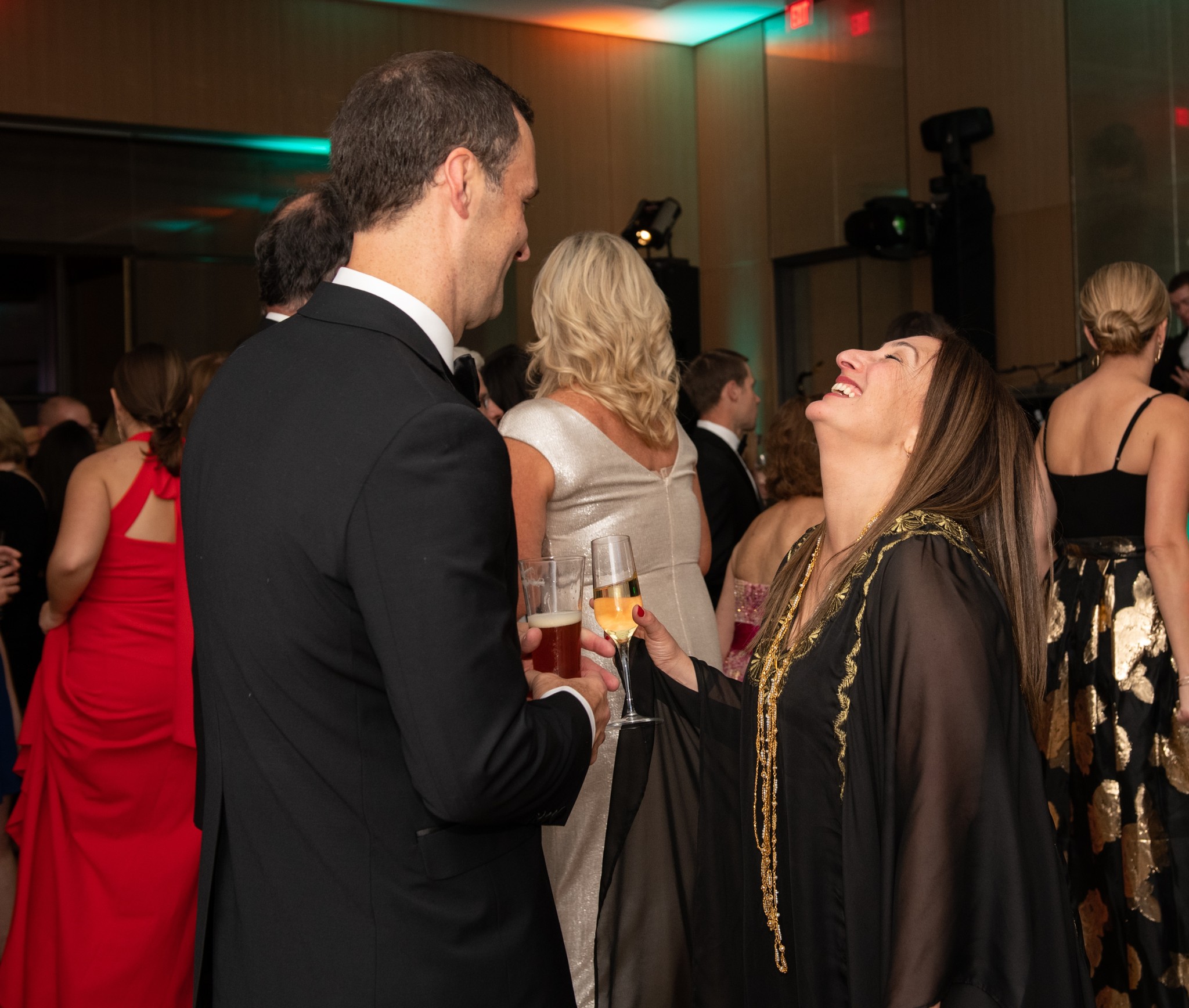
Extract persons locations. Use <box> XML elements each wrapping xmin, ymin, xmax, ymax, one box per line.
<box><xmin>2</xmin><ymin>179</ymin><xmax>354</xmax><ymax>1008</ymax></box>
<box><xmin>475</xmin><ymin>226</ymin><xmax>828</xmax><ymax>1008</ymax></box>
<box><xmin>579</xmin><ymin>328</ymin><xmax>1093</xmax><ymax>1008</ymax></box>
<box><xmin>178</xmin><ymin>47</ymin><xmax>615</xmax><ymax>1007</ymax></box>
<box><xmin>1021</xmin><ymin>259</ymin><xmax>1189</xmax><ymax>1008</ymax></box>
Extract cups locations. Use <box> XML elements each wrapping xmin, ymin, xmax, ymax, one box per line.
<box><xmin>519</xmin><ymin>555</ymin><xmax>585</xmax><ymax>699</ymax></box>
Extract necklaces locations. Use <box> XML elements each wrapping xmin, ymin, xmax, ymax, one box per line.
<box><xmin>754</xmin><ymin>507</ymin><xmax>888</xmax><ymax>974</ymax></box>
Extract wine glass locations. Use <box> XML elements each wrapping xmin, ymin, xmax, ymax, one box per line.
<box><xmin>591</xmin><ymin>534</ymin><xmax>665</xmax><ymax>731</ymax></box>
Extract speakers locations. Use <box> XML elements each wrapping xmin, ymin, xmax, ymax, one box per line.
<box><xmin>646</xmin><ymin>265</ymin><xmax>700</xmax><ymax>438</ymax></box>
